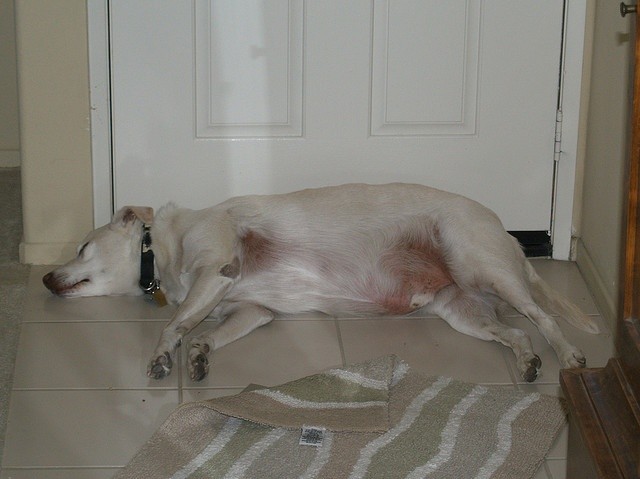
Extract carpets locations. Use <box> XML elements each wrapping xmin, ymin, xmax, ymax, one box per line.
<box><xmin>108</xmin><ymin>351</ymin><xmax>572</xmax><ymax>478</ymax></box>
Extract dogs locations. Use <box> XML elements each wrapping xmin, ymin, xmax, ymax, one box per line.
<box><xmin>41</xmin><ymin>182</ymin><xmax>603</xmax><ymax>384</ymax></box>
